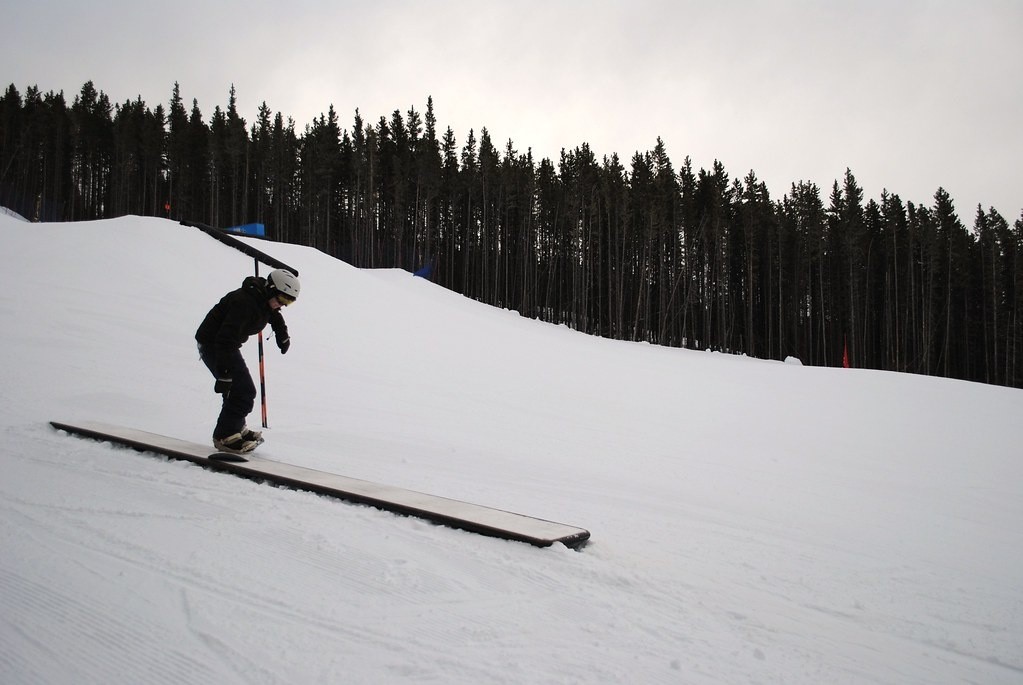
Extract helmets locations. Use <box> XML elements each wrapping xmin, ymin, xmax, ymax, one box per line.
<box><xmin>267</xmin><ymin>269</ymin><xmax>300</xmax><ymax>302</ymax></box>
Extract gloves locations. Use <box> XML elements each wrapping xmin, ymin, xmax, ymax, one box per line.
<box><xmin>214</xmin><ymin>374</ymin><xmax>234</xmax><ymax>393</ymax></box>
<box><xmin>275</xmin><ymin>332</ymin><xmax>290</xmax><ymax>354</ymax></box>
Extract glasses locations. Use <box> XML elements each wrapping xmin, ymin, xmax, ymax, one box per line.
<box><xmin>274</xmin><ymin>296</ymin><xmax>293</xmax><ymax>307</ymax></box>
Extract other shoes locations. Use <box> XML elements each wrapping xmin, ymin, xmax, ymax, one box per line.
<box><xmin>241</xmin><ymin>426</ymin><xmax>262</xmax><ymax>441</ymax></box>
<box><xmin>211</xmin><ymin>432</ymin><xmax>257</xmax><ymax>453</ymax></box>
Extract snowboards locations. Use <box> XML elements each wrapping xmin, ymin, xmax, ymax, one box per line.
<box><xmin>207</xmin><ymin>438</ymin><xmax>265</xmax><ymax>462</ymax></box>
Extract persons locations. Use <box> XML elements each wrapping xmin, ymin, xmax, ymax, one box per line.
<box><xmin>195</xmin><ymin>268</ymin><xmax>300</xmax><ymax>454</ymax></box>
